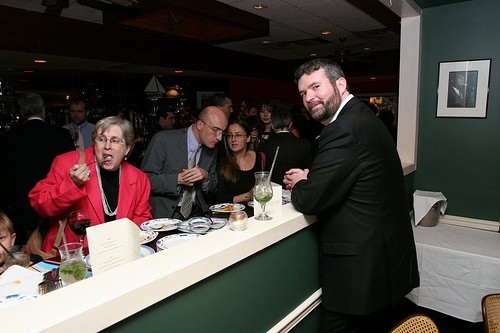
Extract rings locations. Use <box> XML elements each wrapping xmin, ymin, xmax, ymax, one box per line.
<box><xmin>87</xmin><ymin>173</ymin><xmax>89</xmax><ymax>176</ymax></box>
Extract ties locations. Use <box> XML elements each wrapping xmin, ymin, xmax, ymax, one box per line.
<box><xmin>180</xmin><ymin>144</ymin><xmax>201</xmax><ymax>218</ymax></box>
<box><xmin>75</xmin><ymin>126</ymin><xmax>84</xmax><ymax>150</ymax></box>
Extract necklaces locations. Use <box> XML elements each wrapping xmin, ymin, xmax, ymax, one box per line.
<box><xmin>94</xmin><ymin>155</ymin><xmax>122</xmax><ymax>216</ymax></box>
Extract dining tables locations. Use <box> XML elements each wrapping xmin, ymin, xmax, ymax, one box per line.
<box><xmin>48</xmin><ymin>198</ymin><xmax>257</xmax><ymax>275</ymax></box>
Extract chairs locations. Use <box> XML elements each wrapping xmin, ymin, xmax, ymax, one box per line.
<box><xmin>482</xmin><ymin>294</ymin><xmax>500</xmax><ymax>332</ymax></box>
<box><xmin>391</xmin><ymin>314</ymin><xmax>440</xmax><ymax>333</ymax></box>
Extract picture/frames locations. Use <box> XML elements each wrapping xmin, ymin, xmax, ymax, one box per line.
<box><xmin>435</xmin><ymin>58</ymin><xmax>491</xmax><ymax>118</ymax></box>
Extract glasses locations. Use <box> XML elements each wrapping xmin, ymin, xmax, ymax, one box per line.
<box><xmin>96</xmin><ymin>135</ymin><xmax>126</xmax><ymax>145</ymax></box>
<box><xmin>201</xmin><ymin>119</ymin><xmax>227</xmax><ymax>137</ymax></box>
<box><xmin>227</xmin><ymin>133</ymin><xmax>247</xmax><ymax>139</ymax></box>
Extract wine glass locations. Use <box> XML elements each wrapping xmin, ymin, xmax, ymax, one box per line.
<box><xmin>70</xmin><ymin>126</ymin><xmax>79</xmax><ymax>147</ymax></box>
<box><xmin>254</xmin><ymin>171</ymin><xmax>273</xmax><ymax>220</ymax></box>
<box><xmin>69</xmin><ymin>210</ymin><xmax>91</xmax><ymax>257</ymax></box>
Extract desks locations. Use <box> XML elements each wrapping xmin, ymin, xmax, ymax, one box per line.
<box><xmin>401</xmin><ymin>219</ymin><xmax>500</xmax><ymax>322</ymax></box>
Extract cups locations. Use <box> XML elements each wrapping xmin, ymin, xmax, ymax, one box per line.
<box><xmin>3</xmin><ymin>245</ymin><xmax>31</xmax><ymax>268</ymax></box>
<box><xmin>58</xmin><ymin>243</ymin><xmax>87</xmax><ymax>284</ymax></box>
<box><xmin>229</xmin><ymin>211</ymin><xmax>249</xmax><ymax>231</ymax></box>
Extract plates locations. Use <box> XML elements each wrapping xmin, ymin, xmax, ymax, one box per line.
<box><xmin>85</xmin><ymin>217</ymin><xmax>228</xmax><ymax>272</ymax></box>
<box><xmin>209</xmin><ymin>202</ymin><xmax>245</xmax><ymax>213</ymax></box>
<box><xmin>248</xmin><ymin>200</ymin><xmax>286</xmax><ymax>207</ymax></box>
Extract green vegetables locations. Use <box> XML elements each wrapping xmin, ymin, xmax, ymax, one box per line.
<box><xmin>259</xmin><ymin>194</ymin><xmax>272</xmax><ymax>202</ymax></box>
<box><xmin>61</xmin><ymin>260</ymin><xmax>86</xmax><ymax>281</ymax></box>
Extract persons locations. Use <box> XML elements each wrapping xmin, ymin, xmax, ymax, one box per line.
<box><xmin>62</xmin><ymin>96</ymin><xmax>125</xmax><ymax>150</ymax></box>
<box><xmin>283</xmin><ymin>59</ymin><xmax>421</xmax><ymax>333</ymax></box>
<box><xmin>27</xmin><ymin>116</ymin><xmax>153</xmax><ymax>255</ymax></box>
<box><xmin>0</xmin><ymin>212</ymin><xmax>43</xmax><ymax>274</ymax></box>
<box><xmin>0</xmin><ymin>92</ymin><xmax>76</xmax><ymax>245</ymax></box>
<box><xmin>141</xmin><ymin>90</ymin><xmax>397</xmax><ymax>221</ymax></box>
